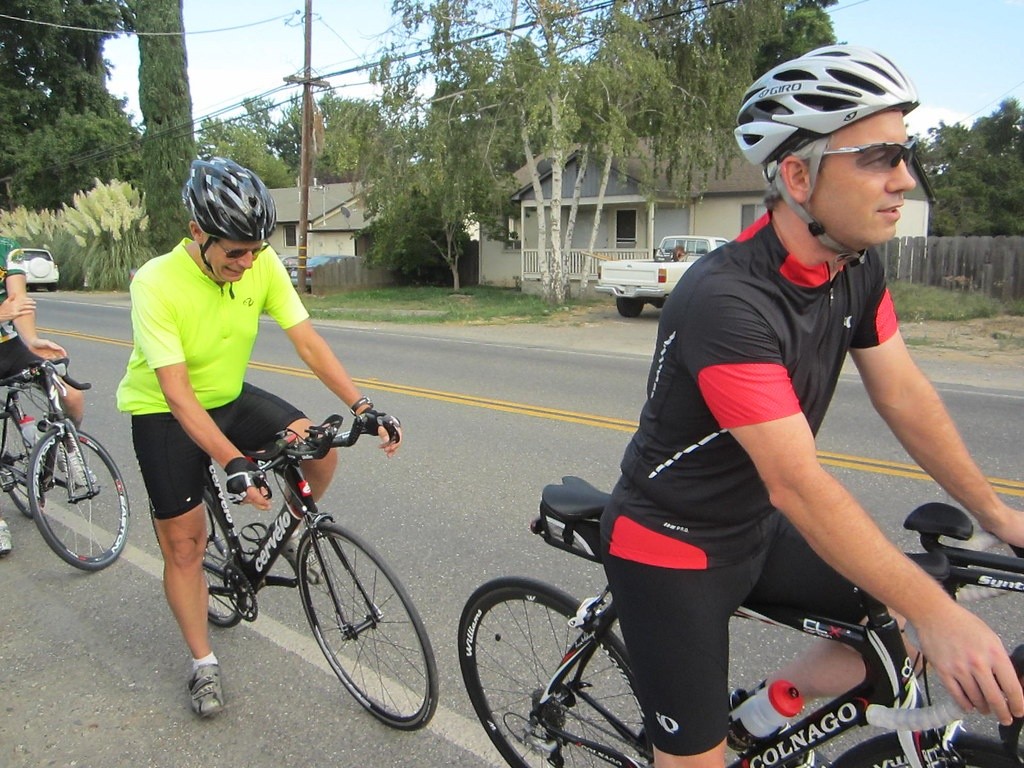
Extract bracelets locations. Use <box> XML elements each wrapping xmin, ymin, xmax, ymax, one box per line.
<box><xmin>351</xmin><ymin>396</ymin><xmax>373</xmax><ymax>417</ymax></box>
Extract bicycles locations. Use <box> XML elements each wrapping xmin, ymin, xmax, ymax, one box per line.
<box><xmin>146</xmin><ymin>402</ymin><xmax>438</xmax><ymax>731</ymax></box>
<box><xmin>0</xmin><ymin>357</ymin><xmax>131</xmax><ymax>571</ymax></box>
<box><xmin>455</xmin><ymin>473</ymin><xmax>1023</xmax><ymax>768</ymax></box>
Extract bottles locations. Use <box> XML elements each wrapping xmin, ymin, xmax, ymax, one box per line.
<box><xmin>34</xmin><ymin>420</ymin><xmax>47</xmax><ymax>445</ymax></box>
<box><xmin>729</xmin><ymin>681</ymin><xmax>804</xmax><ymax>751</ymax></box>
<box><xmin>18</xmin><ymin>413</ymin><xmax>38</xmax><ymax>453</ymax></box>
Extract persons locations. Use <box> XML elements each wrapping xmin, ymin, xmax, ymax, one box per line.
<box><xmin>599</xmin><ymin>43</ymin><xmax>1024</xmax><ymax>768</ymax></box>
<box><xmin>116</xmin><ymin>155</ymin><xmax>403</xmax><ymax>717</ymax></box>
<box><xmin>0</xmin><ymin>237</ymin><xmax>98</xmax><ymax>556</ymax></box>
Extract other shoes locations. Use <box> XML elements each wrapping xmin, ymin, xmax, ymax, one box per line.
<box><xmin>727</xmin><ymin>686</ymin><xmax>833</xmax><ymax>768</ymax></box>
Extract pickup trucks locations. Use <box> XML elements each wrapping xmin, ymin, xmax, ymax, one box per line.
<box><xmin>594</xmin><ymin>234</ymin><xmax>731</xmax><ymax>317</ymax></box>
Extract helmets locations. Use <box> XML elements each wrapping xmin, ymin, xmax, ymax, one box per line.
<box><xmin>181</xmin><ymin>155</ymin><xmax>277</xmax><ymax>242</ymax></box>
<box><xmin>731</xmin><ymin>43</ymin><xmax>920</xmax><ymax>165</ymax></box>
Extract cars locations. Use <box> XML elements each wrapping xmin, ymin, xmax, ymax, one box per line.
<box><xmin>292</xmin><ymin>254</ymin><xmax>354</xmax><ymax>291</ymax></box>
<box><xmin>275</xmin><ymin>254</ymin><xmax>300</xmax><ymax>272</ymax></box>
<box><xmin>2</xmin><ymin>247</ymin><xmax>60</xmax><ymax>292</ymax></box>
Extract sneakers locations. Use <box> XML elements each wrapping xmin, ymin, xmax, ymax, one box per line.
<box><xmin>281</xmin><ymin>531</ymin><xmax>326</xmax><ymax>585</ymax></box>
<box><xmin>57</xmin><ymin>450</ymin><xmax>98</xmax><ymax>485</ymax></box>
<box><xmin>187</xmin><ymin>655</ymin><xmax>225</xmax><ymax>718</ymax></box>
<box><xmin>0</xmin><ymin>519</ymin><xmax>12</xmax><ymax>555</ymax></box>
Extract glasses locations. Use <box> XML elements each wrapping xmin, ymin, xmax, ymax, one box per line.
<box><xmin>799</xmin><ymin>135</ymin><xmax>917</xmax><ymax>173</ymax></box>
<box><xmin>214</xmin><ymin>239</ymin><xmax>270</xmax><ymax>259</ymax></box>
